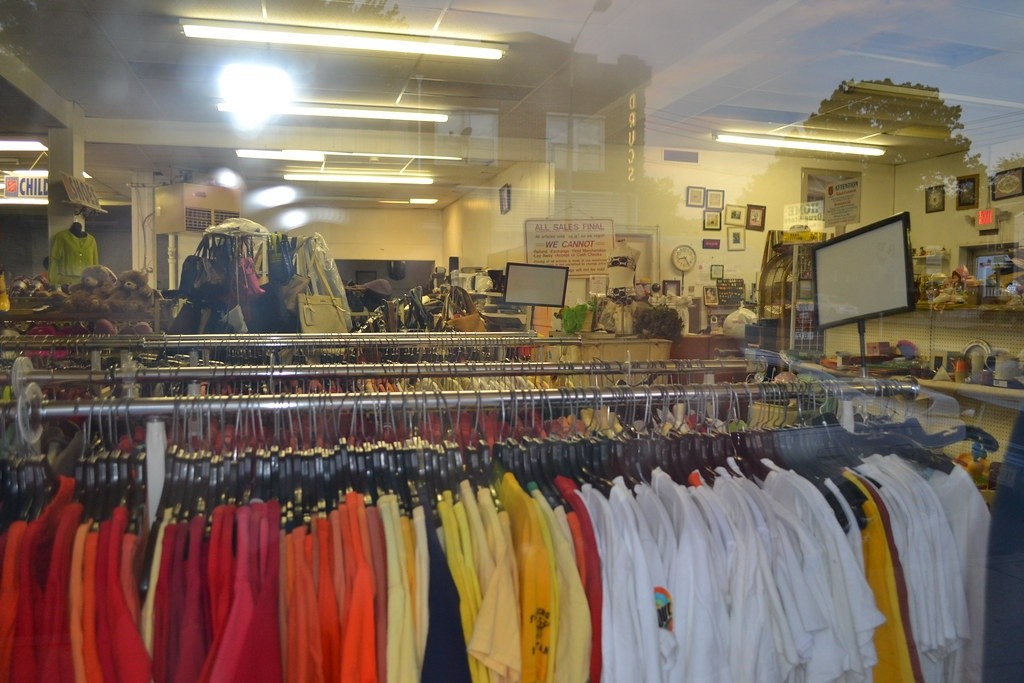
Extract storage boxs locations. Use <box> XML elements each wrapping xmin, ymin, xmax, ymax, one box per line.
<box><xmin>867</xmin><ymin>342</ymin><xmax>889</xmax><ymax>355</ymax></box>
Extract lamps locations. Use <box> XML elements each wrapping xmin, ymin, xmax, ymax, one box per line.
<box><xmin>215</xmin><ymin>99</ymin><xmax>452</xmax><ymax>122</ymax></box>
<box><xmin>964</xmin><ymin>208</ymin><xmax>1012</xmax><ymax>230</ymax></box>
<box><xmin>177</xmin><ymin>18</ymin><xmax>509</xmax><ymax>60</ymax></box>
<box><xmin>713</xmin><ymin>132</ymin><xmax>888</xmax><ymax>157</ymax></box>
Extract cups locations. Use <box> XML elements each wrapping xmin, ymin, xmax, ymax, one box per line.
<box><xmin>971</xmin><ymin>353</ymin><xmax>984</xmax><ymax>372</ymax></box>
<box><xmin>955</xmin><ymin>360</ymin><xmax>967</xmax><ymax>372</ymax></box>
<box><xmin>986</xmin><ymin>354</ymin><xmax>1019</xmax><ymax>381</ymax></box>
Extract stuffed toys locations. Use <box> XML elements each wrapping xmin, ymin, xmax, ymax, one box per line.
<box><xmin>50</xmin><ymin>266</ymin><xmax>155</xmax><ymax>333</ymax></box>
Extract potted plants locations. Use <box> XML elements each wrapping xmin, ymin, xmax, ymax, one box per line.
<box><xmin>633</xmin><ymin>304</ymin><xmax>684</xmax><ymax>347</ymax></box>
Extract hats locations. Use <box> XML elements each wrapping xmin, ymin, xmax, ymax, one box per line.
<box><xmin>364</xmin><ymin>278</ymin><xmax>392</xmax><ymax>298</ymax></box>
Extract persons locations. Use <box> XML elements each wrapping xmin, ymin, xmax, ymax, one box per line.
<box><xmin>606</xmin><ymin>239</ymin><xmax>641</xmax><ymax>297</ymax></box>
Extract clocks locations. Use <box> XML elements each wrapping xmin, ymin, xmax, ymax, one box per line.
<box><xmin>671</xmin><ymin>244</ymin><xmax>697</xmax><ymax>271</ymax></box>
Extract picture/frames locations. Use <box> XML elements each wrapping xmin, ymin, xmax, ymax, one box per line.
<box><xmin>992</xmin><ymin>167</ymin><xmax>1024</xmax><ymax>201</ymax></box>
<box><xmin>499</xmin><ymin>183</ymin><xmax>509</xmax><ymax>215</ymax></box>
<box><xmin>956</xmin><ymin>174</ymin><xmax>979</xmax><ymax>210</ymax></box>
<box><xmin>703</xmin><ymin>264</ymin><xmax>724</xmax><ymax>306</ymax></box>
<box><xmin>925</xmin><ymin>184</ymin><xmax>945</xmax><ymax>213</ymax></box>
<box><xmin>663</xmin><ymin>280</ymin><xmax>680</xmax><ymax>296</ymax></box>
<box><xmin>686</xmin><ymin>186</ymin><xmax>724</xmax><ymax>230</ymax></box>
<box><xmin>725</xmin><ymin>204</ymin><xmax>766</xmax><ymax>251</ymax></box>
<box><xmin>356</xmin><ymin>270</ymin><xmax>377</xmax><ymax>285</ymax></box>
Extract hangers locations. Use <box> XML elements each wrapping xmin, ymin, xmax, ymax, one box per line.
<box><xmin>0</xmin><ymin>336</ymin><xmax>999</xmax><ymax>598</ymax></box>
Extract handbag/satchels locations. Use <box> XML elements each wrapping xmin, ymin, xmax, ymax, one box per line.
<box><xmin>166</xmin><ymin>231</ymin><xmax>347</xmax><ymax>355</ymax></box>
<box><xmin>441</xmin><ymin>287</ymin><xmax>487</xmax><ymax>332</ymax></box>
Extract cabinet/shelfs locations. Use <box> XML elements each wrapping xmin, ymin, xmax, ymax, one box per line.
<box><xmin>434</xmin><ymin>292</ymin><xmax>527</xmax><ymax>325</ymax></box>
<box><xmin>799</xmin><ymin>302</ymin><xmax>1024</xmax><ymax>400</ymax></box>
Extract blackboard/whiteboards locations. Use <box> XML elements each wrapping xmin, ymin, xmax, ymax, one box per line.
<box><xmin>503</xmin><ymin>262</ymin><xmax>570</xmax><ymax>308</ymax></box>
<box><xmin>811</xmin><ymin>211</ymin><xmax>914</xmax><ymax>329</ymax></box>
